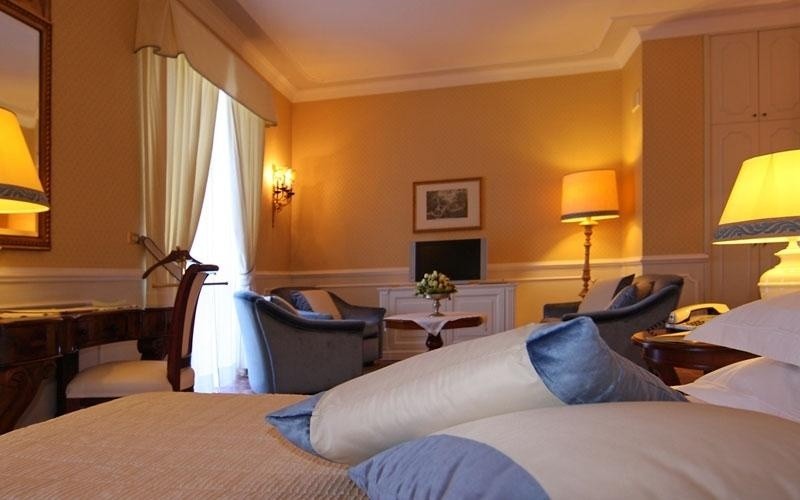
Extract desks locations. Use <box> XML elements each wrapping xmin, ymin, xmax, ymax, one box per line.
<box><xmin>0</xmin><ymin>305</ymin><xmax>175</xmax><ymax>433</ymax></box>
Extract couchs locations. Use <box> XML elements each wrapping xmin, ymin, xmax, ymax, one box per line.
<box><xmin>543</xmin><ymin>273</ymin><xmax>683</xmax><ymax>367</ymax></box>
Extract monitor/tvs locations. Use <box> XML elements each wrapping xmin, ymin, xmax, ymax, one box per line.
<box><xmin>410</xmin><ymin>236</ymin><xmax>486</xmax><ymax>285</ymax></box>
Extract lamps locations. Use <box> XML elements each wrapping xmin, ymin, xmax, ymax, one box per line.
<box><xmin>712</xmin><ymin>149</ymin><xmax>800</xmax><ymax>300</ymax></box>
<box><xmin>0</xmin><ymin>105</ymin><xmax>50</xmax><ymax>214</ymax></box>
<box><xmin>272</xmin><ymin>164</ymin><xmax>295</xmax><ymax>229</ymax></box>
<box><xmin>560</xmin><ymin>169</ymin><xmax>620</xmax><ymax>299</ymax></box>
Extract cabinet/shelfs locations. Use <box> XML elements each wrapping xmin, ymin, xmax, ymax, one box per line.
<box><xmin>703</xmin><ymin>28</ymin><xmax>800</xmax><ymax>124</ymax></box>
<box><xmin>704</xmin><ymin>118</ymin><xmax>800</xmax><ymax>308</ymax></box>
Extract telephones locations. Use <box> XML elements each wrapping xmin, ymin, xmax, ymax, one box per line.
<box><xmin>646</xmin><ymin>303</ymin><xmax>731</xmax><ymax>336</ymax></box>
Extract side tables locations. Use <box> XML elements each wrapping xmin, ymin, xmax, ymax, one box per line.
<box><xmin>386</xmin><ymin>312</ymin><xmax>483</xmax><ymax>352</ymax></box>
<box><xmin>631</xmin><ymin>329</ymin><xmax>760</xmax><ymax>396</ymax></box>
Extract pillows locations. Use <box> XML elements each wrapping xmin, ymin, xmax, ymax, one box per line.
<box><xmin>265</xmin><ymin>316</ymin><xmax>691</xmax><ymax>464</ymax></box>
<box><xmin>346</xmin><ymin>403</ymin><xmax>799</xmax><ymax>500</ymax></box>
<box><xmin>263</xmin><ymin>294</ymin><xmax>299</xmax><ymax>315</ymax></box>
<box><xmin>604</xmin><ymin>280</ymin><xmax>655</xmax><ymax>310</ymax></box>
<box><xmin>684</xmin><ymin>290</ymin><xmax>800</xmax><ymax>368</ymax></box>
<box><xmin>577</xmin><ymin>273</ymin><xmax>634</xmax><ymax>313</ymax></box>
<box><xmin>290</xmin><ymin>289</ymin><xmax>344</xmax><ymax>321</ymax></box>
<box><xmin>670</xmin><ymin>357</ymin><xmax>800</xmax><ymax>423</ymax></box>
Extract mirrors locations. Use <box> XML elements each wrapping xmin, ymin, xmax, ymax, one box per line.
<box><xmin>0</xmin><ymin>1</ymin><xmax>53</xmax><ymax>251</ymax></box>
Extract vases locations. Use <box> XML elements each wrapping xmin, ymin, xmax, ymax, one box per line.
<box><xmin>425</xmin><ymin>293</ymin><xmax>445</xmax><ymax>318</ymax></box>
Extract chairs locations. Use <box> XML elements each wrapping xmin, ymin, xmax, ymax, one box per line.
<box><xmin>271</xmin><ymin>286</ymin><xmax>386</xmax><ymax>366</ymax></box>
<box><xmin>68</xmin><ymin>264</ymin><xmax>219</xmax><ymax>413</ymax></box>
<box><xmin>233</xmin><ymin>290</ymin><xmax>366</xmax><ymax>395</ymax></box>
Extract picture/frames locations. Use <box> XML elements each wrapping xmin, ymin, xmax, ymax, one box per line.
<box><xmin>413</xmin><ymin>176</ymin><xmax>482</xmax><ymax>234</ymax></box>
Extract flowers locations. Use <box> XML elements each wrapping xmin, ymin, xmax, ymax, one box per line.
<box><xmin>414</xmin><ymin>270</ymin><xmax>457</xmax><ymax>297</ymax></box>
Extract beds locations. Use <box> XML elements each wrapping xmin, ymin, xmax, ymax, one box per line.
<box><xmin>0</xmin><ymin>391</ymin><xmax>369</xmax><ymax>500</ymax></box>
